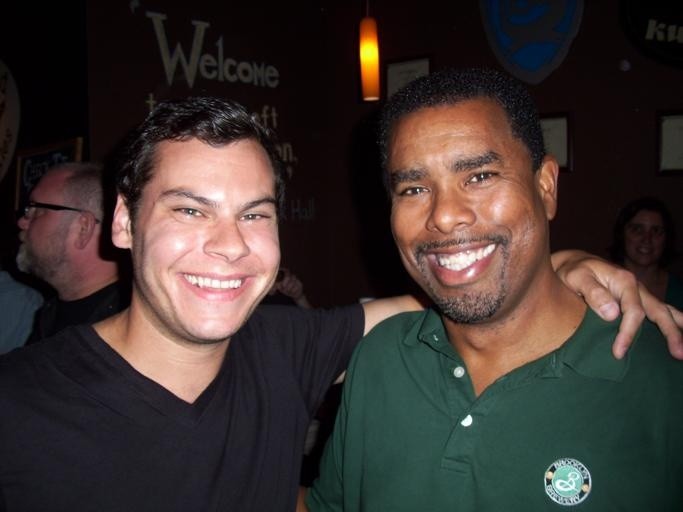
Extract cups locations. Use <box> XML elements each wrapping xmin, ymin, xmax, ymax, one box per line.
<box><xmin>24</xmin><ymin>201</ymin><xmax>101</xmax><ymax>223</ymax></box>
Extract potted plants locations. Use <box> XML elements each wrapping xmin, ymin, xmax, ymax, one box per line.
<box><xmin>656</xmin><ymin>110</ymin><xmax>683</xmax><ymax>176</ymax></box>
<box><xmin>13</xmin><ymin>137</ymin><xmax>83</xmax><ymax>211</ymax></box>
<box><xmin>537</xmin><ymin>111</ymin><xmax>574</xmax><ymax>173</ymax></box>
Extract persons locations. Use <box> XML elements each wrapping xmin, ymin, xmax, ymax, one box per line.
<box><xmin>0</xmin><ymin>270</ymin><xmax>45</xmax><ymax>359</ymax></box>
<box><xmin>14</xmin><ymin>156</ymin><xmax>134</xmax><ymax>350</ymax></box>
<box><xmin>0</xmin><ymin>95</ymin><xmax>681</xmax><ymax>510</ymax></box>
<box><xmin>291</xmin><ymin>60</ymin><xmax>683</xmax><ymax>510</ymax></box>
<box><xmin>609</xmin><ymin>194</ymin><xmax>682</xmax><ymax>315</ymax></box>
<box><xmin>263</xmin><ymin>255</ymin><xmax>319</xmax><ymax>311</ymax></box>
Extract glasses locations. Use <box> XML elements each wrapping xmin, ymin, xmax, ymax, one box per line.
<box><xmin>359</xmin><ymin>0</ymin><xmax>381</xmax><ymax>102</ymax></box>
